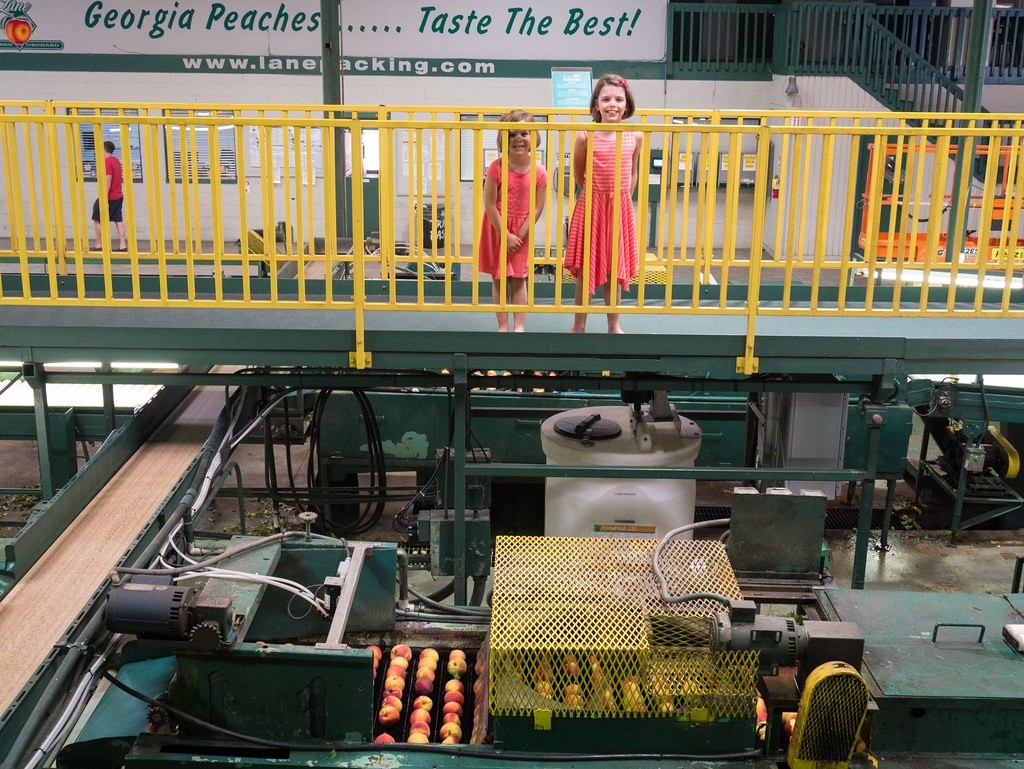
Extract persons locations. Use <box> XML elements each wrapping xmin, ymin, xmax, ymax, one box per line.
<box><xmin>88</xmin><ymin>140</ymin><xmax>129</xmax><ymax>252</ymax></box>
<box><xmin>477</xmin><ymin>107</ymin><xmax>549</xmax><ymax>333</ymax></box>
<box><xmin>563</xmin><ymin>72</ymin><xmax>645</xmax><ymax>334</ymax></box>
